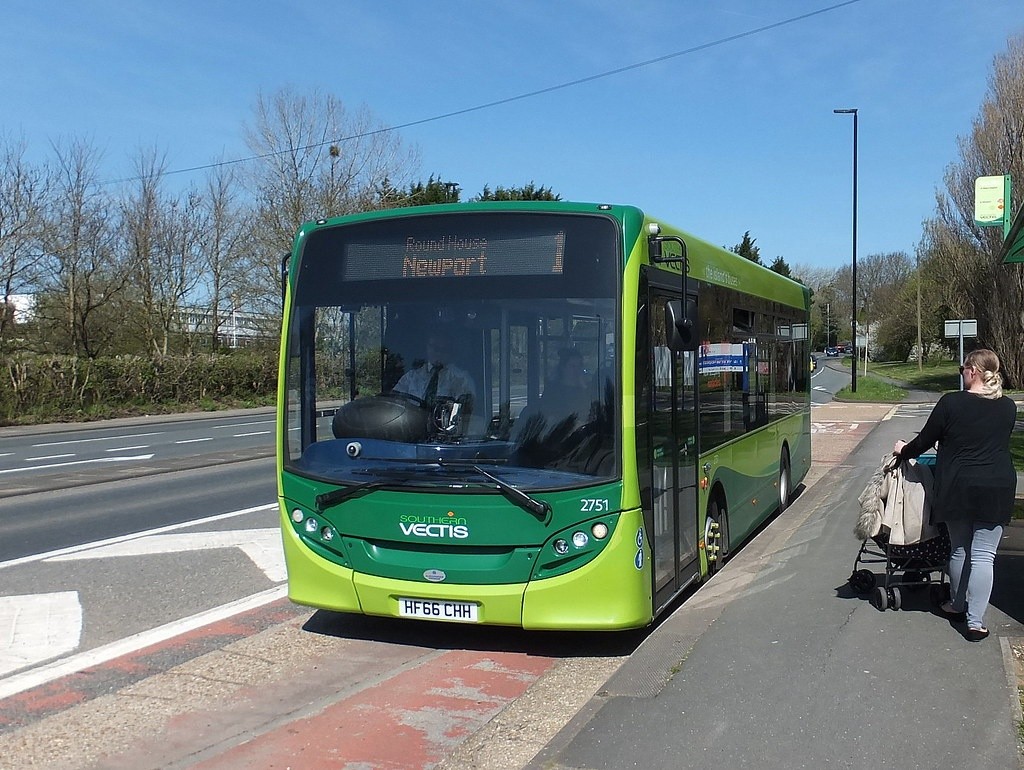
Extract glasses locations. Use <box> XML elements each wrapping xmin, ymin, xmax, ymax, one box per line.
<box><xmin>958</xmin><ymin>364</ymin><xmax>981</xmax><ymax>375</ymax></box>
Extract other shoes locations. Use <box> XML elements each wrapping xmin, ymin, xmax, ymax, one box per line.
<box><xmin>934</xmin><ymin>599</ymin><xmax>967</xmax><ymax>622</ymax></box>
<box><xmin>965</xmin><ymin>624</ymin><xmax>992</xmax><ymax>642</ymax></box>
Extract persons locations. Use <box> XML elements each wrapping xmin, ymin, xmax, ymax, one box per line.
<box><xmin>541</xmin><ymin>349</ymin><xmax>585</xmax><ymax>402</ymax></box>
<box><xmin>894</xmin><ymin>349</ymin><xmax>1017</xmax><ymax>642</ymax></box>
<box><xmin>388</xmin><ymin>331</ymin><xmax>475</xmax><ymax>436</ymax></box>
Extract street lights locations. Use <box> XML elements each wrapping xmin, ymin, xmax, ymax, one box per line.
<box><xmin>834</xmin><ymin>108</ymin><xmax>859</xmax><ymax>393</ymax></box>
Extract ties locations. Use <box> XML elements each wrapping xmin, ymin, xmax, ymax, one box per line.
<box><xmin>424</xmin><ymin>365</ymin><xmax>444</xmax><ymax>408</ymax></box>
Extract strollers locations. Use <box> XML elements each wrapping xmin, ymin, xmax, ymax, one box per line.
<box><xmin>850</xmin><ymin>439</ymin><xmax>951</xmax><ymax>611</ymax></box>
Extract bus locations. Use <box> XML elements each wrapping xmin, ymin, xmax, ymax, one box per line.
<box><xmin>275</xmin><ymin>200</ymin><xmax>812</xmax><ymax>633</ymax></box>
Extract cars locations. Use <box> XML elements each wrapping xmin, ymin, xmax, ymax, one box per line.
<box><xmin>826</xmin><ymin>347</ymin><xmax>838</xmax><ymax>357</ymax></box>
<box><xmin>837</xmin><ymin>344</ymin><xmax>845</xmax><ymax>353</ymax></box>
<box><xmin>844</xmin><ymin>345</ymin><xmax>853</xmax><ymax>354</ymax></box>
<box><xmin>810</xmin><ymin>355</ymin><xmax>819</xmax><ymax>369</ymax></box>
<box><xmin>809</xmin><ymin>357</ymin><xmax>816</xmax><ymax>373</ymax></box>
